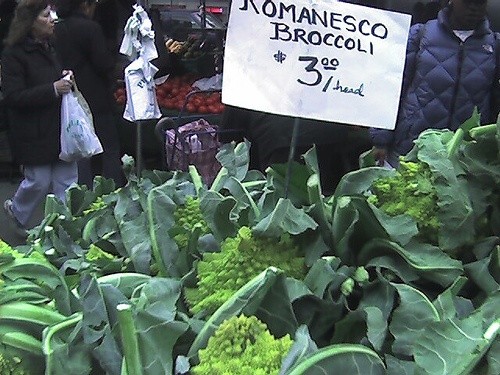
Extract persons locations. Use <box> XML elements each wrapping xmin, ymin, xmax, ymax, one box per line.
<box><xmin>218</xmin><ymin>103</ymin><xmax>349</xmax><ymax>186</ymax></box>
<box><xmin>2</xmin><ymin>0</ymin><xmax>79</xmax><ymax>239</ymax></box>
<box><xmin>51</xmin><ymin>0</ymin><xmax>131</xmax><ymax>190</ymax></box>
<box><xmin>369</xmin><ymin>0</ymin><xmax>500</xmax><ymax>166</ymax></box>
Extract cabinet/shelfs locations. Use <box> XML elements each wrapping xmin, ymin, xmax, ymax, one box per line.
<box><xmin>149</xmin><ymin>9</ymin><xmax>228</xmax><ymax>70</ymax></box>
<box><xmin>115</xmin><ymin>105</ymin><xmax>222</xmax><ymax>181</ymax></box>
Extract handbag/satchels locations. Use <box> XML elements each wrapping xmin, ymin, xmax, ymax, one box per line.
<box><xmin>71</xmin><ymin>91</ymin><xmax>94</xmax><ymax>128</ymax></box>
<box><xmin>165</xmin><ymin>118</ymin><xmax>222</xmax><ymax>187</ymax></box>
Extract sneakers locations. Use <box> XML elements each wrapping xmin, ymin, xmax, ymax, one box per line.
<box><xmin>3</xmin><ymin>199</ymin><xmax>28</xmax><ymax>237</ymax></box>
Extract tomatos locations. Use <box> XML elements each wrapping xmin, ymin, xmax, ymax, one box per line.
<box><xmin>112</xmin><ymin>70</ymin><xmax>236</xmax><ymax>115</ymax></box>
